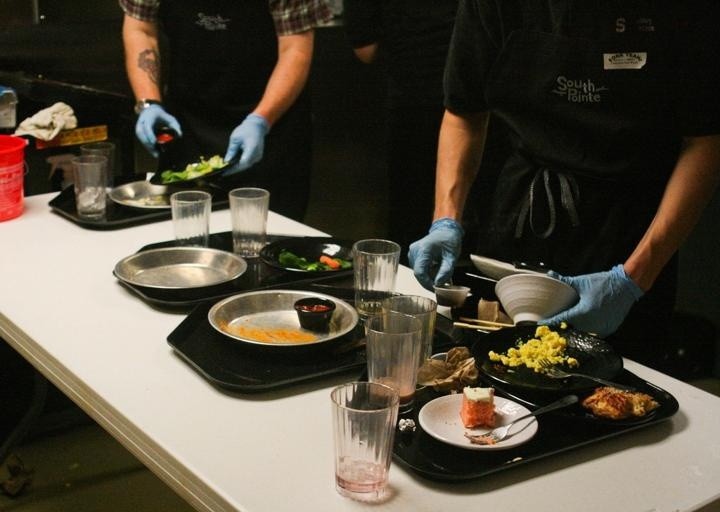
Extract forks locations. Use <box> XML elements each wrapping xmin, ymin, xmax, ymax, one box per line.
<box><xmin>539</xmin><ymin>357</ymin><xmax>639</xmax><ymax>396</ymax></box>
<box><xmin>466</xmin><ymin>395</ymin><xmax>578</xmax><ymax>444</ymax></box>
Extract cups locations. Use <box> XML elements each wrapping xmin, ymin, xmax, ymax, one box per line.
<box><xmin>228</xmin><ymin>187</ymin><xmax>271</xmax><ymax>260</ymax></box>
<box><xmin>352</xmin><ymin>239</ymin><xmax>401</xmax><ymax>327</ymax></box>
<box><xmin>433</xmin><ymin>285</ymin><xmax>471</xmax><ymax>314</ymax></box>
<box><xmin>331</xmin><ymin>380</ymin><xmax>400</xmax><ymax>503</ymax></box>
<box><xmin>365</xmin><ymin>313</ymin><xmax>423</xmax><ymax>415</ymax></box>
<box><xmin>170</xmin><ymin>189</ymin><xmax>212</xmax><ymax>248</ymax></box>
<box><xmin>71</xmin><ymin>155</ymin><xmax>110</xmax><ymax>220</ymax></box>
<box><xmin>293</xmin><ymin>297</ymin><xmax>336</xmax><ymax>329</ymax></box>
<box><xmin>153</xmin><ymin>128</ymin><xmax>177</xmax><ymax>153</ymax></box>
<box><xmin>381</xmin><ymin>295</ymin><xmax>437</xmax><ymax>362</ymax></box>
<box><xmin>80</xmin><ymin>142</ymin><xmax>116</xmax><ymax>206</ymax></box>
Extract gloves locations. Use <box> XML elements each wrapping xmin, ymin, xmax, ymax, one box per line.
<box><xmin>536</xmin><ymin>265</ymin><xmax>645</xmax><ymax>337</ymax></box>
<box><xmin>136</xmin><ymin>105</ymin><xmax>183</xmax><ymax>156</ymax></box>
<box><xmin>409</xmin><ymin>218</ymin><xmax>467</xmax><ymax>292</ymax></box>
<box><xmin>225</xmin><ymin>114</ymin><xmax>271</xmax><ymax>177</ymax></box>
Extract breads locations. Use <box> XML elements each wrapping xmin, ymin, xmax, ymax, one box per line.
<box><xmin>460</xmin><ymin>386</ymin><xmax>497</xmax><ymax>429</ymax></box>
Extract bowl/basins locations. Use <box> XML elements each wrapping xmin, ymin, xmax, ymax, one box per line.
<box><xmin>493</xmin><ymin>273</ymin><xmax>578</xmax><ymax>325</ymax></box>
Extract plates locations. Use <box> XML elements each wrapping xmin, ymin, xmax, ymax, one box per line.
<box><xmin>258</xmin><ymin>236</ymin><xmax>354</xmax><ymax>279</ymax></box>
<box><xmin>109</xmin><ymin>182</ymin><xmax>169</xmax><ymax>213</ymax></box>
<box><xmin>476</xmin><ymin>323</ymin><xmax>623</xmax><ymax>401</ymax></box>
<box><xmin>208</xmin><ymin>289</ymin><xmax>358</xmax><ymax>349</ymax></box>
<box><xmin>428</xmin><ymin>353</ymin><xmax>449</xmax><ymax>361</ymax></box>
<box><xmin>470</xmin><ymin>253</ymin><xmax>542</xmax><ymax>280</ymax></box>
<box><xmin>115</xmin><ymin>247</ymin><xmax>249</xmax><ymax>290</ymax></box>
<box><xmin>572</xmin><ymin>388</ymin><xmax>659</xmax><ymax>425</ymax></box>
<box><xmin>417</xmin><ymin>394</ymin><xmax>538</xmax><ymax>453</ymax></box>
<box><xmin>149</xmin><ymin>152</ymin><xmax>241</xmax><ymax>187</ymax></box>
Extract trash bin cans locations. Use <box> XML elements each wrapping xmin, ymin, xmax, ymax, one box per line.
<box><xmin>0</xmin><ymin>67</ymin><xmax>136</xmax><ymax>196</ymax></box>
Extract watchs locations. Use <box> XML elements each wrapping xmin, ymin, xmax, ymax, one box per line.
<box><xmin>133</xmin><ymin>101</ymin><xmax>165</xmax><ymax>115</ymax></box>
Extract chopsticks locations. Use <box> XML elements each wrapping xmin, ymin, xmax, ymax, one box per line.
<box><xmin>453</xmin><ymin>316</ymin><xmax>516</xmax><ymax>333</ymax></box>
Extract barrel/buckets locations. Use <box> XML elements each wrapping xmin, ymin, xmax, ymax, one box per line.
<box><xmin>0</xmin><ymin>134</ymin><xmax>29</xmax><ymax>223</ymax></box>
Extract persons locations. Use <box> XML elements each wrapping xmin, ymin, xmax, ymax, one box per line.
<box><xmin>121</xmin><ymin>0</ymin><xmax>347</xmax><ymax>225</ymax></box>
<box><xmin>407</xmin><ymin>0</ymin><xmax>719</xmax><ymax>382</ymax></box>
